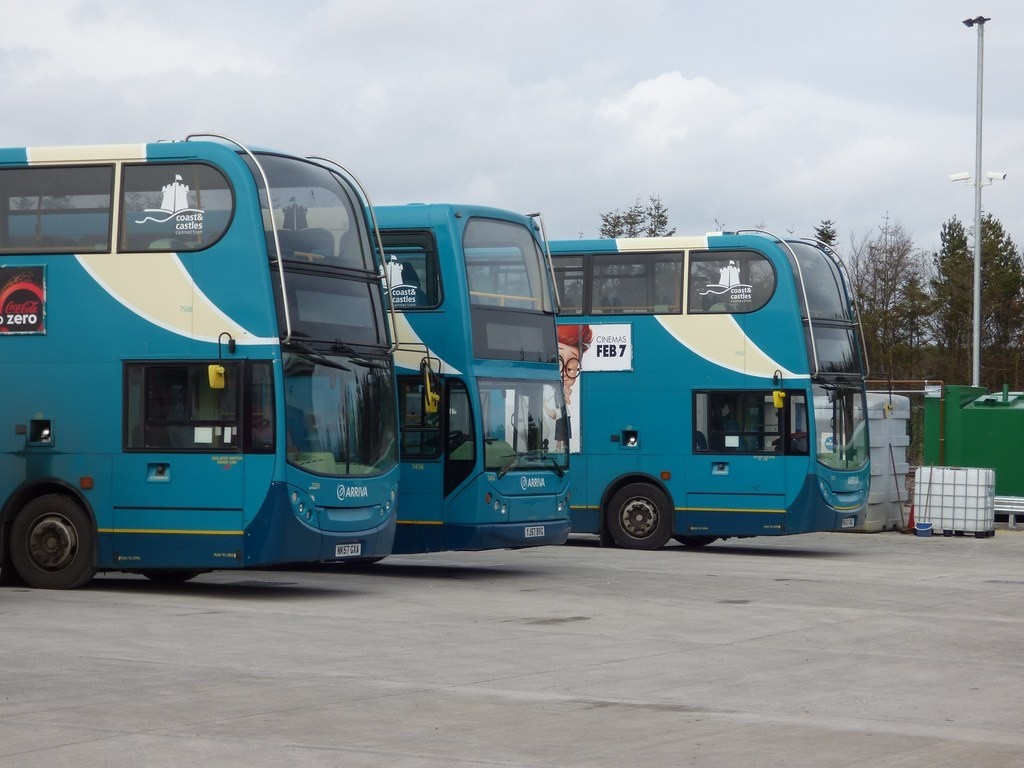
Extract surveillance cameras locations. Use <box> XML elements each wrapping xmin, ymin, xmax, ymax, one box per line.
<box><xmin>987</xmin><ymin>172</ymin><xmax>1007</xmax><ymax>179</ymax></box>
<box><xmin>950</xmin><ymin>172</ymin><xmax>972</xmax><ymax>181</ymax></box>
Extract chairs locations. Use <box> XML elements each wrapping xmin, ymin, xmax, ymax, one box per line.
<box><xmin>1</xmin><ymin>225</ymin><xmax>777</xmax><ymax>459</ymax></box>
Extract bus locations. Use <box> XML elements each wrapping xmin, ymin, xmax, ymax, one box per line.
<box><xmin>1</xmin><ymin>133</ymin><xmax>444</xmax><ymax>589</ymax></box>
<box><xmin>155</xmin><ymin>202</ymin><xmax>580</xmax><ymax>565</ymax></box>
<box><xmin>389</xmin><ymin>228</ymin><xmax>871</xmax><ymax>551</ymax></box>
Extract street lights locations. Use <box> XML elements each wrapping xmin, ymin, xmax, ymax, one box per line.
<box><xmin>963</xmin><ymin>16</ymin><xmax>993</xmax><ymax>389</ymax></box>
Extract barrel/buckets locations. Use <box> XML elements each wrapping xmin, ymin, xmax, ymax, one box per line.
<box><xmin>915</xmin><ymin>522</ymin><xmax>932</xmax><ymax>536</ymax></box>
